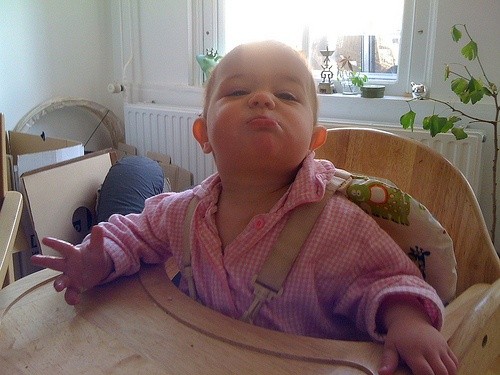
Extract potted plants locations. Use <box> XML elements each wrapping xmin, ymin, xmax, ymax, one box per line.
<box><xmin>335</xmin><ymin>54</ymin><xmax>368</xmax><ymax>97</ymax></box>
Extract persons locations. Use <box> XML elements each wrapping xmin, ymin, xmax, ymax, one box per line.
<box><xmin>31</xmin><ymin>40</ymin><xmax>458</xmax><ymax>375</ymax></box>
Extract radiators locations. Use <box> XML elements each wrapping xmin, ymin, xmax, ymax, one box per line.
<box><xmin>120</xmin><ymin>103</ymin><xmax>484</xmax><ymax>207</ymax></box>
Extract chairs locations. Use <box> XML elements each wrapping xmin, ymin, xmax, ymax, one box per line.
<box><xmin>0</xmin><ymin>127</ymin><xmax>500</xmax><ymax>375</ymax></box>
